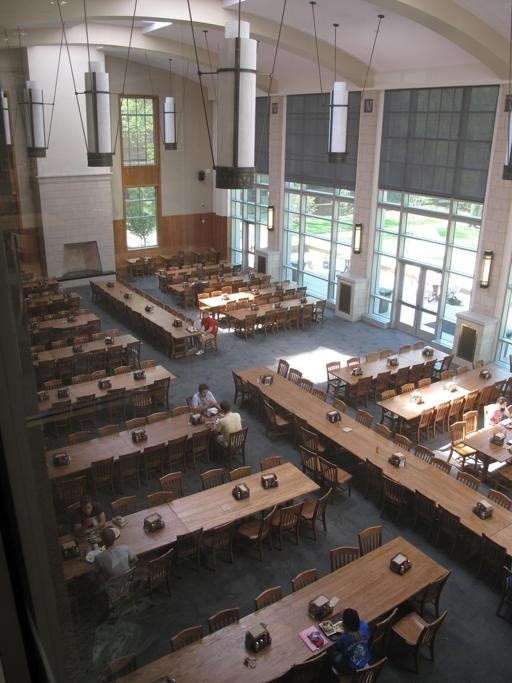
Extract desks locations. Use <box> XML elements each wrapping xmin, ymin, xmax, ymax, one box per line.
<box><xmin>226</xmin><ymin>296</ymin><xmax>323</xmax><ymax>321</ymax></box>
<box><xmin>198</xmin><ymin>280</ymin><xmax>301</xmax><ymax>308</ymax></box>
<box><xmin>460</xmin><ymin>416</ymin><xmax>512</xmax><ymax>463</ymax></box>
<box><xmin>327</xmin><ymin>346</ymin><xmax>453</xmax><ymax>386</ymax></box>
<box><xmin>30</xmin><ymin>334</ymin><xmax>140</xmax><ymax>363</ymax></box>
<box><xmin>113</xmin><ymin>534</ymin><xmax>451</xmax><ymax>682</ymax></box>
<box><xmin>36</xmin><ymin>363</ymin><xmax>176</xmax><ymax>414</ymax></box>
<box><xmin>93</xmin><ymin>280</ymin><xmax>201</xmax><ymax>360</ymax></box>
<box><xmin>32</xmin><ymin>312</ymin><xmax>103</xmax><ymax>335</ymax></box>
<box><xmin>236</xmin><ymin>366</ymin><xmax>512</xmax><ymax>557</ymax></box>
<box><xmin>44</xmin><ymin>412</ymin><xmax>246</xmax><ymax>482</ymax></box>
<box><xmin>55</xmin><ymin>461</ymin><xmax>322</xmax><ymax>582</ymax></box>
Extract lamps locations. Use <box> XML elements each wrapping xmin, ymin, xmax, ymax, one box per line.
<box><xmin>267</xmin><ymin>205</ymin><xmax>275</xmax><ymax>231</ymax></box>
<box><xmin>2</xmin><ymin>20</ymin><xmax>68</xmax><ymax>159</ymax></box>
<box><xmin>143</xmin><ymin>50</ymin><xmax>190</xmax><ymax>151</ymax></box>
<box><xmin>477</xmin><ymin>249</ymin><xmax>494</xmax><ymax>289</ymax></box>
<box><xmin>1</xmin><ymin>92</ymin><xmax>21</xmax><ymax>149</ymax></box>
<box><xmin>308</xmin><ymin>1</ymin><xmax>385</xmax><ymax>164</ymax></box>
<box><xmin>56</xmin><ymin>0</ymin><xmax>140</xmax><ymax>168</ymax></box>
<box><xmin>352</xmin><ymin>222</ymin><xmax>362</xmax><ymax>255</ymax></box>
<box><xmin>186</xmin><ymin>0</ymin><xmax>289</xmax><ymax>190</ymax></box>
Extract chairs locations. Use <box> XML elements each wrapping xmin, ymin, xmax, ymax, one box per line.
<box><xmin>44</xmin><ymin>378</ymin><xmax>63</xmax><ymax>390</ymax></box>
<box><xmin>347</xmin><ymin>357</ymin><xmax>360</xmax><ymax>367</ymax></box>
<box><xmin>136</xmin><ymin>259</ymin><xmax>145</xmax><ymax>277</ymax></box>
<box><xmin>61</xmin><ymin>475</ymin><xmax>87</xmax><ymax>507</ymax></box>
<box><xmin>431</xmin><ymin>400</ymin><xmax>452</xmax><ymax>435</ymax></box>
<box><xmin>146</xmin><ymin>491</ymin><xmax>174</xmax><ymax>508</ymax></box>
<box><xmin>364</xmin><ymin>457</ymin><xmax>383</xmax><ymax>507</ymax></box>
<box><xmin>271</xmin><ymin>501</ymin><xmax>305</xmax><ymax>551</ymax></box>
<box><xmin>418</xmin><ymin>378</ymin><xmax>431</xmax><ymax>389</ymax></box>
<box><xmin>338</xmin><ymin>656</ymin><xmax>388</xmax><ymax>683</ymax></box>
<box><xmin>496</xmin><ymin>565</ymin><xmax>512</xmax><ymax>623</ymax></box>
<box><xmin>51</xmin><ymin>340</ymin><xmax>67</xmax><ymax>349</ymax></box>
<box><xmin>67</xmin><ymin>431</ymin><xmax>91</xmax><ymax>445</ymax></box>
<box><xmin>369</xmin><ymin>608</ymin><xmax>399</xmax><ymax>656</ymax></box>
<box><xmin>208</xmin><ymin>607</ymin><xmax>241</xmax><ymax>634</ymax></box>
<box><xmin>276</xmin><ymin>359</ymin><xmax>289</xmax><ymax>378</ymax></box>
<box><xmin>89</xmin><ymin>348</ymin><xmax>106</xmax><ymax>372</ymax></box>
<box><xmin>413</xmin><ymin>568</ymin><xmax>454</xmax><ymax>619</ymax></box>
<box><xmin>502</xmin><ymin>377</ymin><xmax>512</xmax><ymax>398</ymax></box>
<box><xmin>139</xmin><ymin>360</ymin><xmax>155</xmax><ymax>369</ymax></box>
<box><xmin>400</xmin><ymin>383</ymin><xmax>416</xmax><ymax>393</ymax></box>
<box><xmin>225</xmin><ymin>300</ymin><xmax>238</xmax><ymax>329</ymax></box>
<box><xmin>125</xmin><ymin>417</ymin><xmax>146</xmax><ymax>430</ymax></box>
<box><xmin>189</xmin><ymin>428</ymin><xmax>210</xmax><ymax>464</ymax></box>
<box><xmin>333</xmin><ymin>398</ymin><xmax>347</xmax><ymax>414</ymax></box>
<box><xmin>144</xmin><ymin>442</ymin><xmax>166</xmax><ymax>481</ymax></box>
<box><xmin>237</xmin><ymin>503</ymin><xmax>278</xmax><ymax>562</ymax></box>
<box><xmin>171</xmin><ymin>406</ymin><xmax>190</xmax><ymax>418</ymax></box>
<box><xmin>254</xmin><ymin>286</ymin><xmax>307</xmax><ymax>306</ymax></box>
<box><xmin>389</xmin><ymin>366</ymin><xmax>411</xmax><ymax>390</ymax></box>
<box><xmin>440</xmin><ymin>370</ymin><xmax>456</xmax><ymax>380</ymax></box>
<box><xmin>299</xmin><ymin>426</ymin><xmax>328</xmax><ymax>454</ymax></box>
<box><xmin>147</xmin><ymin>412</ymin><xmax>169</xmax><ymax>424</ymax></box>
<box><xmin>414</xmin><ymin>444</ymin><xmax>435</xmax><ymax>465</ymax></box>
<box><xmin>381</xmin><ymin>474</ymin><xmax>410</xmax><ymax>526</ymax></box>
<box><xmin>127</xmin><ymin>340</ymin><xmax>141</xmax><ymax>364</ymax></box>
<box><xmin>455</xmin><ymin>470</ymin><xmax>481</xmax><ymax>491</ymax></box>
<box><xmin>347</xmin><ymin>376</ymin><xmax>374</xmax><ymax>409</ymax></box>
<box><xmin>255</xmin><ymin>310</ymin><xmax>277</xmax><ymax>336</ymax></box>
<box><xmin>106</xmin><ymin>345</ymin><xmax>123</xmax><ymax>373</ymax></box>
<box><xmin>39</xmin><ymin>360</ymin><xmax>56</xmax><ymax>390</ymax></box>
<box><xmin>167</xmin><ymin>434</ymin><xmax>189</xmax><ymax>473</ymax></box>
<box><xmin>260</xmin><ymin>456</ymin><xmax>281</xmax><ymax>471</ymax></box>
<box><xmin>215</xmin><ymin>427</ymin><xmax>248</xmax><ymax>465</ymax></box>
<box><xmin>73</xmin><ymin>328</ymin><xmax>121</xmax><ymax>345</ymax></box>
<box><xmin>238</xmin><ymin>287</ymin><xmax>249</xmax><ymax>292</ymax></box>
<box><xmin>210</xmin><ymin>291</ymin><xmax>223</xmax><ymax>298</ymax></box>
<box><xmin>479</xmin><ymin>384</ymin><xmax>494</xmax><ymax>405</ymax></box>
<box><xmin>287</xmin><ymin>368</ymin><xmax>302</xmax><ymax>384</ymax></box>
<box><xmin>429</xmin><ymin>457</ymin><xmax>452</xmax><ymax>475</ymax></box>
<box><xmin>369</xmin><ymin>370</ymin><xmax>392</xmax><ymax>404</ymax></box>
<box><xmin>254</xmin><ymin>585</ymin><xmax>282</xmax><ymax>611</ymax></box>
<box><xmin>222</xmin><ymin>286</ymin><xmax>233</xmax><ymax>294</ymax></box>
<box><xmin>110</xmin><ymin>495</ymin><xmax>137</xmax><ymax>517</ymax></box>
<box><xmin>399</xmin><ymin>343</ymin><xmax>412</xmax><ymax>354</ymax></box>
<box><xmin>27</xmin><ymin>308</ymin><xmax>89</xmax><ymax>324</ymax></box>
<box><xmin>392</xmin><ymin>433</ymin><xmax>413</xmax><ymax>452</ymax></box>
<box><xmin>197</xmin><ymin>293</ymin><xmax>210</xmax><ymax>318</ymax></box>
<box><xmin>433</xmin><ymin>354</ymin><xmax>454</xmax><ymax>377</ymax></box>
<box><xmin>412</xmin><ymin>341</ymin><xmax>426</xmax><ymax>350</ymax></box>
<box><xmin>301</xmin><ymin>304</ymin><xmax>313</xmax><ymax>327</ymax></box>
<box><xmin>97</xmin><ymin>424</ymin><xmax>120</xmax><ymax>437</ymax></box>
<box><xmin>105</xmin><ymin>565</ymin><xmax>137</xmax><ymax>619</ymax></box>
<box><xmin>381</xmin><ymin>389</ymin><xmax>399</xmax><ymax>430</ymax></box>
<box><xmin>106</xmin><ymin>387</ymin><xmax>127</xmax><ymax>423</ymax></box>
<box><xmin>447</xmin><ymin>395</ymin><xmax>466</xmax><ymax>431</ymax></box>
<box><xmin>374</xmin><ymin>422</ymin><xmax>392</xmax><ymax>439</ymax></box>
<box><xmin>160</xmin><ymin>526</ymin><xmax>204</xmax><ymax>583</ymax></box>
<box><xmin>91</xmin><ymin>370</ymin><xmax>107</xmax><ymax>380</ymax></box>
<box><xmin>72</xmin><ymin>373</ymin><xmax>90</xmax><ymax>384</ymax></box>
<box><xmin>484</xmin><ymin>404</ymin><xmax>497</xmax><ymax>427</ymax></box>
<box><xmin>135</xmin><ymin>548</ymin><xmax>175</xmax><ymax>600</ymax></box>
<box><xmin>463</xmin><ymin>388</ymin><xmax>480</xmax><ymax>410</ymax></box>
<box><xmin>296</xmin><ymin>379</ymin><xmax>314</xmax><ymax>393</ymax></box>
<box><xmin>51</xmin><ymin>399</ymin><xmax>73</xmax><ymax>436</ymax></box>
<box><xmin>423</xmin><ymin>358</ymin><xmax>437</xmax><ymax>378</ymax></box>
<box><xmin>273</xmin><ymin>306</ymin><xmax>288</xmax><ymax>333</ymax></box>
<box><xmin>229</xmin><ymin>466</ymin><xmax>252</xmax><ymax>481</ymax></box>
<box><xmin>462</xmin><ymin>410</ymin><xmax>478</xmax><ymax>436</ymax></box>
<box><xmin>118</xmin><ymin>450</ymin><xmax>141</xmax><ymax>491</ymax></box>
<box><xmin>391</xmin><ymin>610</ymin><xmax>449</xmax><ymax>675</ymax></box>
<box><xmin>199</xmin><ymin>520</ymin><xmax>237</xmax><ymax>571</ymax></box>
<box><xmin>104</xmin><ymin>654</ymin><xmax>137</xmax><ymax>680</ymax></box>
<box><xmin>91</xmin><ymin>456</ymin><xmax>116</xmax><ymax>497</ymax></box>
<box><xmin>408</xmin><ymin>362</ymin><xmax>425</xmax><ymax>382</ymax></box>
<box><xmin>319</xmin><ymin>456</ymin><xmax>353</xmax><ymax>497</ymax></box>
<box><xmin>487</xmin><ymin>379</ymin><xmax>507</xmax><ymax>403</ymax></box>
<box><xmin>457</xmin><ymin>365</ymin><xmax>468</xmax><ymax>374</ymax></box>
<box><xmin>237</xmin><ymin>298</ymin><xmax>249</xmax><ymax>308</ymax></box>
<box><xmin>151</xmin><ymin>377</ymin><xmax>170</xmax><ymax>410</ymax></box>
<box><xmin>287</xmin><ymin>305</ymin><xmax>301</xmax><ymax>331</ymax></box>
<box><xmin>412</xmin><ymin>488</ymin><xmax>436</xmax><ymax>540</ymax></box>
<box><xmin>72</xmin><ymin>351</ymin><xmax>89</xmax><ymax>375</ymax></box>
<box><xmin>381</xmin><ymin>350</ymin><xmax>393</xmax><ymax>360</ymax></box>
<box><xmin>131</xmin><ymin>388</ymin><xmax>152</xmax><ymax>416</ymax></box>
<box><xmin>300</xmin><ymin>486</ymin><xmax>333</xmax><ymax>541</ymax></box>
<box><xmin>232</xmin><ymin>369</ymin><xmax>255</xmax><ymax>408</ymax></box>
<box><xmin>159</xmin><ymin>471</ymin><xmax>183</xmax><ymax>500</ymax></box>
<box><xmin>57</xmin><ymin>356</ymin><xmax>74</xmax><ymax>383</ymax></box>
<box><xmin>298</xmin><ymin>443</ymin><xmax>334</xmax><ymax>474</ymax></box>
<box><xmin>357</xmin><ymin>525</ymin><xmax>383</xmax><ymax>557</ymax></box>
<box><xmin>237</xmin><ymin>313</ymin><xmax>257</xmax><ymax>340</ymax></box>
<box><xmin>205</xmin><ymin>321</ymin><xmax>218</xmax><ymax>353</ymax></box>
<box><xmin>290</xmin><ymin>568</ymin><xmax>317</xmax><ymax>592</ymax></box>
<box><xmin>329</xmin><ymin>546</ymin><xmax>360</xmax><ymax>572</ymax></box>
<box><xmin>76</xmin><ymin>394</ymin><xmax>97</xmax><ymax>430</ymax></box>
<box><xmin>374</xmin><ymin>364</ymin><xmax>511</xmax><ymax>422</ymax></box>
<box><xmin>407</xmin><ymin>409</ymin><xmax>434</xmax><ymax>443</ymax></box>
<box><xmin>477</xmin><ymin>532</ymin><xmax>507</xmax><ymax>591</ymax></box>
<box><xmin>264</xmin><ymin>399</ymin><xmax>289</xmax><ymax>440</ymax></box>
<box><xmin>169</xmin><ymin>624</ymin><xmax>204</xmax><ymax>651</ymax></box>
<box><xmin>326</xmin><ymin>361</ymin><xmax>348</xmax><ymax>397</ymax></box>
<box><xmin>434</xmin><ymin>503</ymin><xmax>461</xmax><ymax>555</ymax></box>
<box><xmin>487</xmin><ymin>489</ymin><xmax>512</xmax><ymax>510</ymax></box>
<box><xmin>200</xmin><ymin>468</ymin><xmax>225</xmax><ymax>490</ymax></box>
<box><xmin>313</xmin><ymin>300</ymin><xmax>326</xmax><ymax>324</ymax></box>
<box><xmin>366</xmin><ymin>352</ymin><xmax>381</xmax><ymax>362</ymax></box>
<box><xmin>312</xmin><ymin>389</ymin><xmax>328</xmax><ymax>402</ymax></box>
<box><xmin>446</xmin><ymin>421</ymin><xmax>478</xmax><ymax>471</ymax></box>
<box><xmin>112</xmin><ymin>366</ymin><xmax>131</xmax><ymax>375</ymax></box>
<box><xmin>355</xmin><ymin>409</ymin><xmax>374</xmax><ymax>428</ymax></box>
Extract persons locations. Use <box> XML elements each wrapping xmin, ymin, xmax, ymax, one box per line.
<box><xmin>488</xmin><ymin>397</ymin><xmax>511</xmax><ymax>426</ymax></box>
<box><xmin>187</xmin><ymin>311</ymin><xmax>218</xmax><ymax>356</ymax></box>
<box><xmin>91</xmin><ymin>528</ymin><xmax>138</xmax><ymax>581</ymax></box>
<box><xmin>328</xmin><ymin>609</ymin><xmax>371</xmax><ymax>674</ymax></box>
<box><xmin>71</xmin><ymin>496</ymin><xmax>106</xmax><ymax>535</ymax></box>
<box><xmin>191</xmin><ymin>384</ymin><xmax>218</xmax><ymax>412</ymax></box>
<box><xmin>210</xmin><ymin>400</ymin><xmax>245</xmax><ymax>448</ymax></box>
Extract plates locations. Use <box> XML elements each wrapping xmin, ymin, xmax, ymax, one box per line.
<box><xmin>319</xmin><ymin>619</ymin><xmax>336</xmax><ymax>636</ymax></box>
<box><xmin>86</xmin><ymin>550</ymin><xmax>109</xmax><ymax>563</ymax></box>
<box><xmin>207</xmin><ymin>407</ymin><xmax>218</xmax><ymax>414</ymax></box>
<box><xmin>109</xmin><ymin>528</ymin><xmax>120</xmax><ymax>537</ymax></box>
<box><xmin>333</xmin><ymin>620</ymin><xmax>348</xmax><ymax>634</ymax></box>
